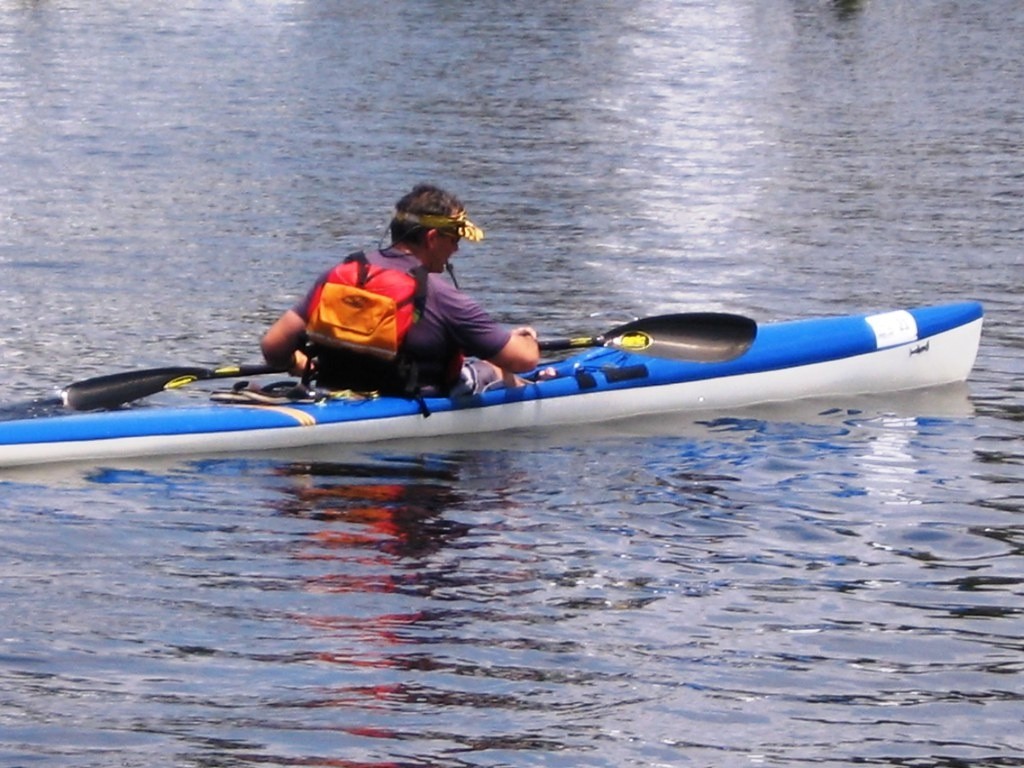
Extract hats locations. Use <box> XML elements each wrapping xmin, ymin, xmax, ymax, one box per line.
<box><xmin>395</xmin><ymin>206</ymin><xmax>485</xmax><ymax>242</ymax></box>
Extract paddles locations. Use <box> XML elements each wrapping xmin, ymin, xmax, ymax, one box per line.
<box><xmin>60</xmin><ymin>311</ymin><xmax>758</xmax><ymax>413</ymax></box>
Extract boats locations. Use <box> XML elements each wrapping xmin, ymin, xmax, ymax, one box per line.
<box><xmin>0</xmin><ymin>300</ymin><xmax>985</xmax><ymax>475</ymax></box>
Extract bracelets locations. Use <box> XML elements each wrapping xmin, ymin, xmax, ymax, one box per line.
<box><xmin>519</xmin><ymin>329</ymin><xmax>533</xmax><ymax>338</ymax></box>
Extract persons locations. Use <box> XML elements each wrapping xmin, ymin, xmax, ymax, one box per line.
<box><xmin>260</xmin><ymin>184</ymin><xmax>538</xmax><ymax>399</ymax></box>
